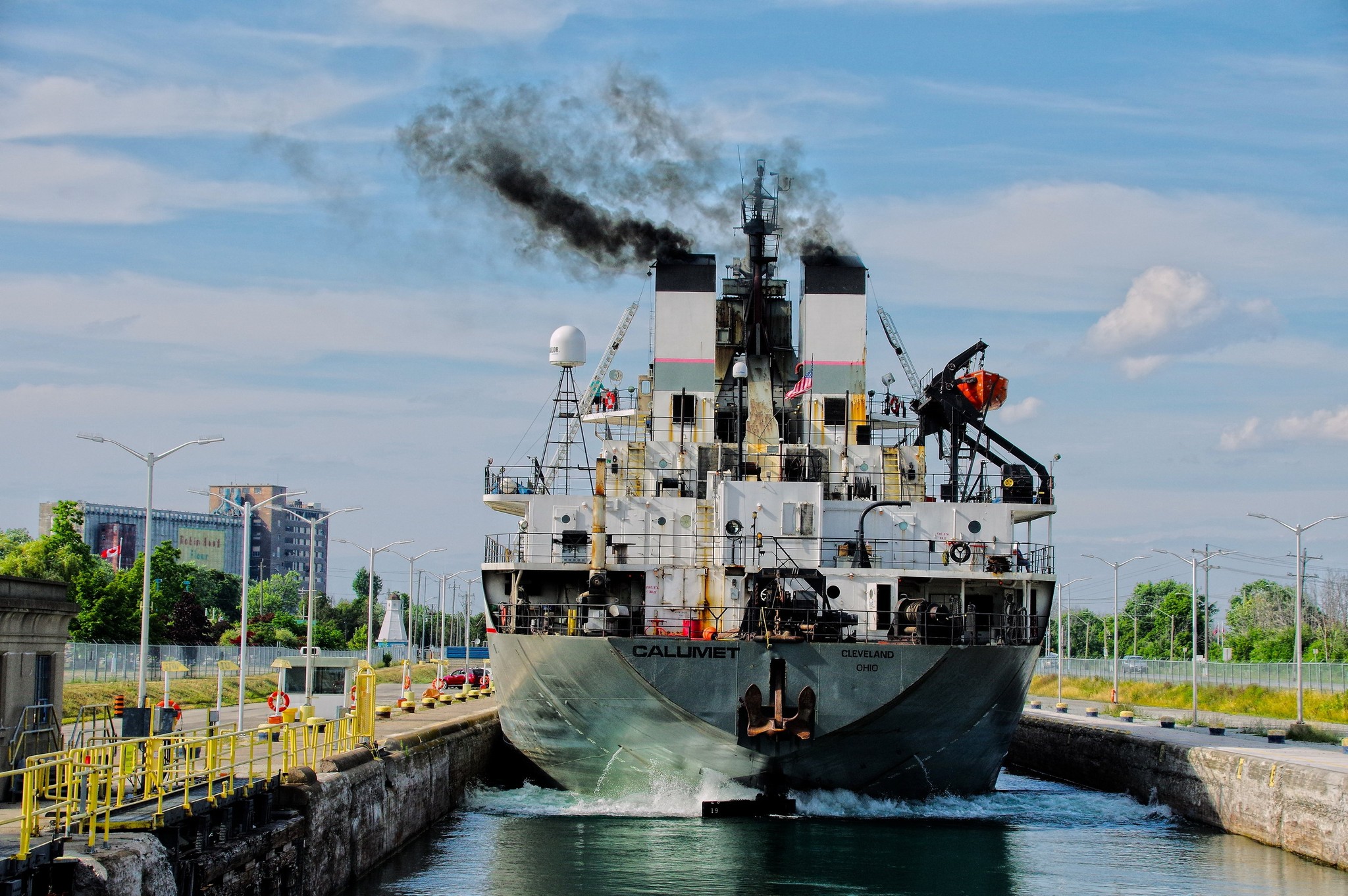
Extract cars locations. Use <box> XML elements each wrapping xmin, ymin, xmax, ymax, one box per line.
<box><xmin>1041</xmin><ymin>656</ymin><xmax>1058</xmax><ymax>668</ymax></box>
<box><xmin>442</xmin><ymin>667</ymin><xmax>489</xmax><ymax>689</ymax></box>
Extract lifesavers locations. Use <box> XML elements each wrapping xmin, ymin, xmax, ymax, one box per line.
<box><xmin>950</xmin><ymin>542</ymin><xmax>971</xmax><ymax>563</ymax></box>
<box><xmin>605</xmin><ymin>392</ymin><xmax>615</xmax><ymax>408</ymax></box>
<box><xmin>432</xmin><ymin>678</ymin><xmax>444</xmax><ymax>689</ymax></box>
<box><xmin>501</xmin><ymin>608</ymin><xmax>506</xmax><ymax>626</ymax></box>
<box><xmin>268</xmin><ymin>691</ymin><xmax>290</xmax><ymax>712</ymax></box>
<box><xmin>480</xmin><ymin>676</ymin><xmax>489</xmax><ymax>685</ymax></box>
<box><xmin>156</xmin><ymin>701</ymin><xmax>181</xmax><ymax>723</ymax></box>
<box><xmin>890</xmin><ymin>397</ymin><xmax>899</xmax><ymax>414</ymax></box>
<box><xmin>351</xmin><ymin>685</ymin><xmax>366</xmax><ymax>701</ymax></box>
<box><xmin>1110</xmin><ymin>690</ymin><xmax>1115</xmax><ymax>702</ymax></box>
<box><xmin>405</xmin><ymin>675</ymin><xmax>410</xmax><ymax>689</ymax></box>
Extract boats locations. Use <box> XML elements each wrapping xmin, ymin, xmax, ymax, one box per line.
<box><xmin>481</xmin><ymin>143</ymin><xmax>1058</xmax><ymax>817</ymax></box>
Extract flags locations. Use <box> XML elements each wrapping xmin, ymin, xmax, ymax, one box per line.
<box><xmin>101</xmin><ymin>545</ymin><xmax>120</xmax><ymax>559</ymax></box>
<box><xmin>784</xmin><ymin>363</ymin><xmax>813</xmax><ymax>401</ymax></box>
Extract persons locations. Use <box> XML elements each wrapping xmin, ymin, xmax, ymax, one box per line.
<box><xmin>500</xmin><ymin>602</ymin><xmax>507</xmax><ymax>634</ymax></box>
<box><xmin>1013</xmin><ymin>540</ymin><xmax>1031</xmax><ymax>573</ymax></box>
<box><xmin>590</xmin><ymin>375</ymin><xmax>608</xmax><ymax>413</ymax></box>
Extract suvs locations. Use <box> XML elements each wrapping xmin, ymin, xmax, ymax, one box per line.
<box><xmin>1121</xmin><ymin>655</ymin><xmax>1149</xmax><ymax>674</ymax></box>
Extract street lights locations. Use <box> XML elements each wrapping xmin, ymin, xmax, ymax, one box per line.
<box><xmin>1054</xmin><ymin>578</ymin><xmax>1092</xmax><ymax>655</ymax></box>
<box><xmin>1176</xmin><ymin>593</ymin><xmax>1208</xmax><ymax>662</ymax></box>
<box><xmin>1111</xmin><ymin>607</ymin><xmax>1136</xmax><ymax>655</ymax></box>
<box><xmin>1152</xmin><ymin>549</ymin><xmax>1238</xmax><ymax>726</ymax></box>
<box><xmin>1248</xmin><ymin>512</ymin><xmax>1348</xmax><ymax>725</ymax></box>
<box><xmin>1080</xmin><ymin>553</ymin><xmax>1152</xmax><ymax>705</ymax></box>
<box><xmin>1140</xmin><ymin>605</ymin><xmax>1174</xmax><ymax>661</ymax></box>
<box><xmin>331</xmin><ymin>538</ymin><xmax>495</xmax><ymax>698</ymax></box>
<box><xmin>1068</xmin><ymin>614</ymin><xmax>1089</xmax><ymax>656</ymax></box>
<box><xmin>1082</xmin><ymin>612</ymin><xmax>1108</xmax><ymax>660</ymax></box>
<box><xmin>76</xmin><ymin>434</ymin><xmax>226</xmax><ymax>794</ymax></box>
<box><xmin>263</xmin><ymin>505</ymin><xmax>363</xmax><ymax>704</ymax></box>
<box><xmin>190</xmin><ymin>491</ymin><xmax>308</xmax><ymax>735</ymax></box>
